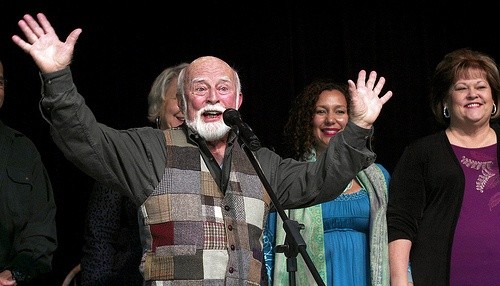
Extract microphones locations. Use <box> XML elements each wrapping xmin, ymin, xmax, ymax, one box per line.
<box><xmin>223</xmin><ymin>108</ymin><xmax>261</xmax><ymax>151</ymax></box>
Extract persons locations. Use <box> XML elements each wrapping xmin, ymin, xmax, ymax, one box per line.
<box><xmin>386</xmin><ymin>47</ymin><xmax>500</xmax><ymax>286</ymax></box>
<box><xmin>11</xmin><ymin>11</ymin><xmax>393</xmax><ymax>286</ymax></box>
<box><xmin>78</xmin><ymin>60</ymin><xmax>193</xmax><ymax>286</ymax></box>
<box><xmin>262</xmin><ymin>78</ymin><xmax>414</xmax><ymax>286</ymax></box>
<box><xmin>0</xmin><ymin>60</ymin><xmax>59</xmax><ymax>286</ymax></box>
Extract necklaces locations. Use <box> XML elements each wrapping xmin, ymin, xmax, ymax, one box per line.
<box><xmin>343</xmin><ymin>179</ymin><xmax>354</xmax><ymax>193</ymax></box>
